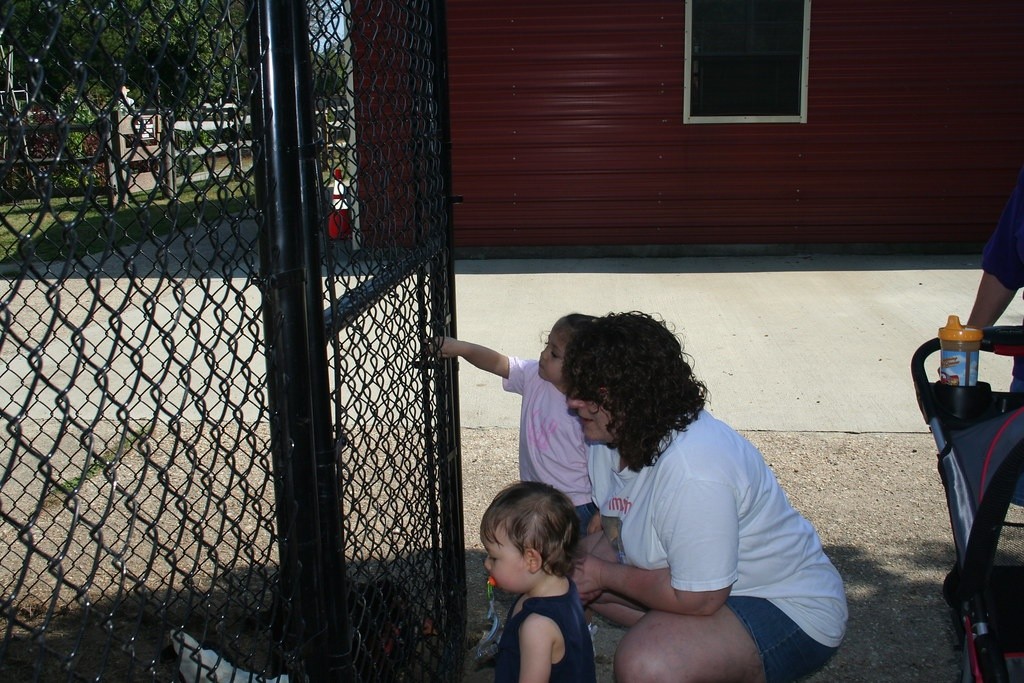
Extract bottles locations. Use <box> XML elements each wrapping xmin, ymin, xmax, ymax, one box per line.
<box><xmin>938</xmin><ymin>316</ymin><xmax>983</xmax><ymax>386</ymax></box>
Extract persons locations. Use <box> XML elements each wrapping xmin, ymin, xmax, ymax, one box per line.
<box><xmin>559</xmin><ymin>313</ymin><xmax>850</xmax><ymax>683</ymax></box>
<box><xmin>424</xmin><ymin>315</ymin><xmax>598</xmax><ymax>539</ymax></box>
<box><xmin>480</xmin><ymin>482</ymin><xmax>597</xmax><ymax>683</ymax></box>
<box><xmin>966</xmin><ymin>165</ymin><xmax>1024</xmax><ymax>528</ymax></box>
<box><xmin>117</xmin><ymin>85</ymin><xmax>135</xmax><ymax>117</ymax></box>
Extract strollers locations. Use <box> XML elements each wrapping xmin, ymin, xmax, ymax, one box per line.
<box><xmin>908</xmin><ymin>322</ymin><xmax>1024</xmax><ymax>683</ymax></box>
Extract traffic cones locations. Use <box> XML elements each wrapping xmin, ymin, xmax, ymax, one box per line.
<box><xmin>328</xmin><ymin>168</ymin><xmax>352</xmax><ymax>238</ymax></box>
